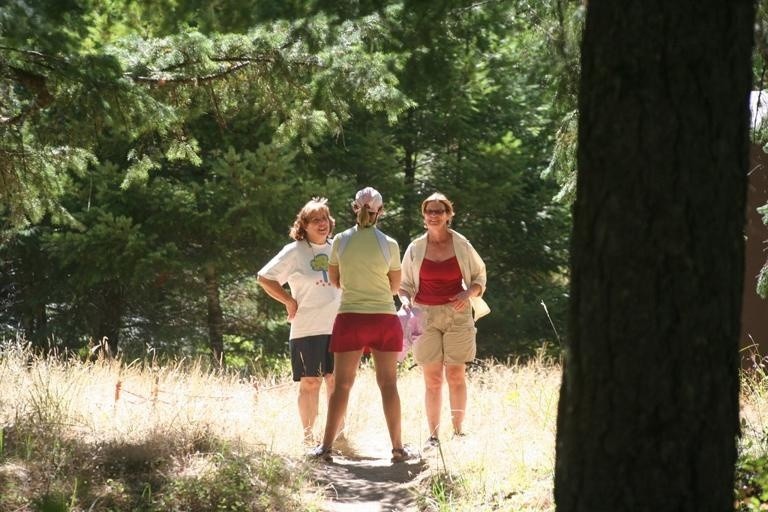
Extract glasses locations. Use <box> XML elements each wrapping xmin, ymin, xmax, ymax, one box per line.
<box><xmin>424</xmin><ymin>209</ymin><xmax>447</xmax><ymax>216</ymax></box>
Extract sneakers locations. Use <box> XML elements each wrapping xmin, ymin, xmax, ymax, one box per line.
<box><xmin>424</xmin><ymin>435</ymin><xmax>439</xmax><ymax>452</ymax></box>
<box><xmin>451</xmin><ymin>432</ymin><xmax>467</xmax><ymax>440</ymax></box>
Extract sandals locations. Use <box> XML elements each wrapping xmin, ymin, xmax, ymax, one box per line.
<box><xmin>305</xmin><ymin>445</ymin><xmax>333</xmax><ymax>464</ymax></box>
<box><xmin>390</xmin><ymin>448</ymin><xmax>412</xmax><ymax>462</ymax></box>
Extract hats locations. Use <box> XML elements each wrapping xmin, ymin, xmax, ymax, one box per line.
<box><xmin>355</xmin><ymin>186</ymin><xmax>383</xmax><ymax>214</ymax></box>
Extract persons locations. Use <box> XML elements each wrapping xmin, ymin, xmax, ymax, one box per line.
<box><xmin>398</xmin><ymin>192</ymin><xmax>492</xmax><ymax>453</ymax></box>
<box><xmin>301</xmin><ymin>187</ymin><xmax>417</xmax><ymax>461</ymax></box>
<box><xmin>256</xmin><ymin>198</ymin><xmax>352</xmax><ymax>458</ymax></box>
